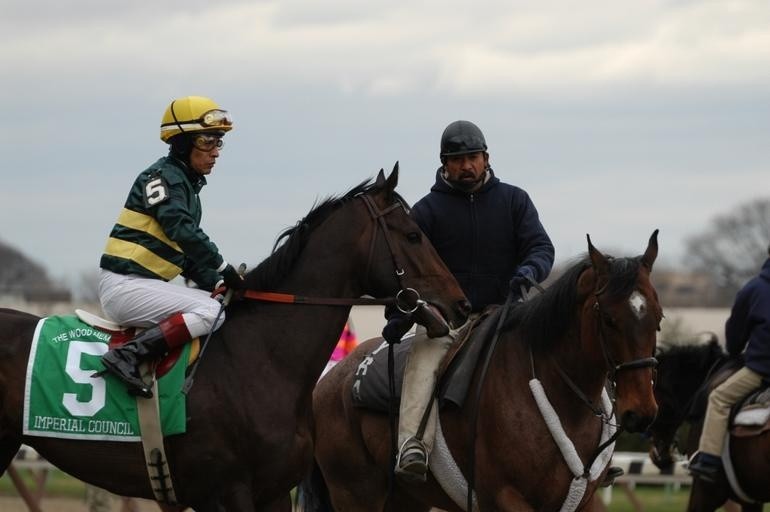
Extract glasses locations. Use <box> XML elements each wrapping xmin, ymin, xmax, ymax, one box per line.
<box><xmin>172</xmin><ymin>100</ymin><xmax>221</xmax><ymax>151</ymax></box>
<box><xmin>161</xmin><ymin>108</ymin><xmax>231</xmax><ymax>126</ymax></box>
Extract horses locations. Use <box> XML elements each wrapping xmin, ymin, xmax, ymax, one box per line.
<box><xmin>0</xmin><ymin>162</ymin><xmax>472</xmax><ymax>511</ymax></box>
<box><xmin>641</xmin><ymin>332</ymin><xmax>770</xmax><ymax>511</ymax></box>
<box><xmin>301</xmin><ymin>229</ymin><xmax>666</xmax><ymax>512</ymax></box>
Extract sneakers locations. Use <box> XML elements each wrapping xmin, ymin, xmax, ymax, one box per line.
<box><xmin>398</xmin><ymin>454</ymin><xmax>427</xmax><ymax>481</ymax></box>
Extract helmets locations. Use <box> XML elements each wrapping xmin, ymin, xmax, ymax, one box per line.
<box><xmin>161</xmin><ymin>97</ymin><xmax>233</xmax><ymax>143</ymax></box>
<box><xmin>440</xmin><ymin>122</ymin><xmax>486</xmax><ymax>155</ymax></box>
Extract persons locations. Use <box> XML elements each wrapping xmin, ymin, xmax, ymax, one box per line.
<box><xmin>381</xmin><ymin>120</ymin><xmax>555</xmax><ymax>483</ymax></box>
<box><xmin>97</xmin><ymin>97</ymin><xmax>244</xmax><ymax>401</ymax></box>
<box><xmin>687</xmin><ymin>242</ymin><xmax>769</xmax><ymax>485</ymax></box>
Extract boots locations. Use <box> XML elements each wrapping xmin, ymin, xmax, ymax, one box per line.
<box><xmin>100</xmin><ymin>315</ymin><xmax>192</xmax><ymax>397</ymax></box>
<box><xmin>688</xmin><ymin>452</ymin><xmax>720</xmax><ymax>510</ymax></box>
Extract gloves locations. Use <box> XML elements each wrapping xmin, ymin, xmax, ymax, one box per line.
<box><xmin>382</xmin><ymin>320</ymin><xmax>405</xmax><ymax>344</ymax></box>
<box><xmin>512</xmin><ymin>265</ymin><xmax>538</xmax><ymax>294</ymax></box>
<box><xmin>218</xmin><ymin>265</ymin><xmax>242</xmax><ymax>296</ymax></box>
